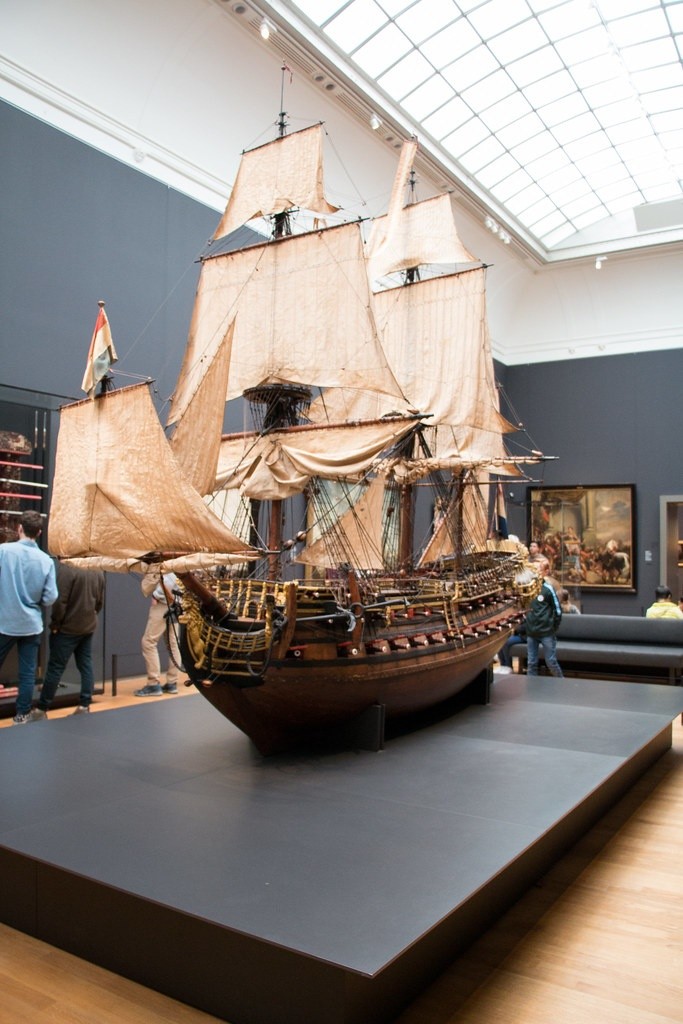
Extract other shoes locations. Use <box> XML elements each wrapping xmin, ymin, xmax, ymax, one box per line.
<box><xmin>67</xmin><ymin>706</ymin><xmax>89</xmax><ymax>716</ymax></box>
<box><xmin>21</xmin><ymin>708</ymin><xmax>47</xmax><ymax>724</ymax></box>
<box><xmin>13</xmin><ymin>713</ymin><xmax>28</xmax><ymax>724</ymax></box>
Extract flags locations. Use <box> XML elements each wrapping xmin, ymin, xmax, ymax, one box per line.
<box><xmin>81</xmin><ymin>308</ymin><xmax>118</xmax><ymax>399</ymax></box>
<box><xmin>489</xmin><ymin>482</ymin><xmax>508</xmax><ymax>539</ymax></box>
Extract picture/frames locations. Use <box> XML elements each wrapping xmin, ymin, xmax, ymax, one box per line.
<box><xmin>525</xmin><ymin>482</ymin><xmax>639</xmax><ymax>596</ymax></box>
<box><xmin>305</xmin><ymin>474</ymin><xmax>411</xmax><ymax>588</ymax></box>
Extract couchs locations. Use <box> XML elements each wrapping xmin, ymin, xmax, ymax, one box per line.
<box><xmin>515</xmin><ymin>614</ymin><xmax>683</xmax><ymax>684</ymax></box>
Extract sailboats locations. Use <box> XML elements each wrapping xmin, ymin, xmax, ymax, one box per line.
<box><xmin>43</xmin><ymin>62</ymin><xmax>560</xmax><ymax>751</ymax></box>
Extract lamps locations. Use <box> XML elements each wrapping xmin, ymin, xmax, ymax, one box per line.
<box><xmin>371</xmin><ymin>119</ymin><xmax>380</xmax><ymax>130</ymax></box>
<box><xmin>485</xmin><ymin>218</ymin><xmax>512</xmax><ymax>244</ymax></box>
<box><xmin>260</xmin><ymin>24</ymin><xmax>269</xmax><ymax>39</ymax></box>
<box><xmin>596</xmin><ymin>258</ymin><xmax>602</xmax><ymax>269</ymax></box>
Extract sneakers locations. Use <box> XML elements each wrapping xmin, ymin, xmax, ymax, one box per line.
<box><xmin>161</xmin><ymin>682</ymin><xmax>177</xmax><ymax>693</ymax></box>
<box><xmin>134</xmin><ymin>685</ymin><xmax>163</xmax><ymax>696</ymax></box>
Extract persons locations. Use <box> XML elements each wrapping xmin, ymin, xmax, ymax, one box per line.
<box><xmin>28</xmin><ymin>561</ymin><xmax>106</xmax><ymax>723</ymax></box>
<box><xmin>497</xmin><ymin>543</ymin><xmax>580</xmax><ymax>677</ymax></box>
<box><xmin>646</xmin><ymin>585</ymin><xmax>683</xmax><ymax>619</ymax></box>
<box><xmin>0</xmin><ymin>510</ymin><xmax>59</xmax><ymax>718</ymax></box>
<box><xmin>135</xmin><ymin>574</ymin><xmax>182</xmax><ymax>697</ymax></box>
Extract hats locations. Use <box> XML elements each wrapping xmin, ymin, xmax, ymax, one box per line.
<box><xmin>556</xmin><ymin>589</ymin><xmax>568</xmax><ymax>601</ymax></box>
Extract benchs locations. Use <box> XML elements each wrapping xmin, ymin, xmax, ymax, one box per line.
<box><xmin>509</xmin><ymin>641</ymin><xmax>683</xmax><ymax>686</ymax></box>
